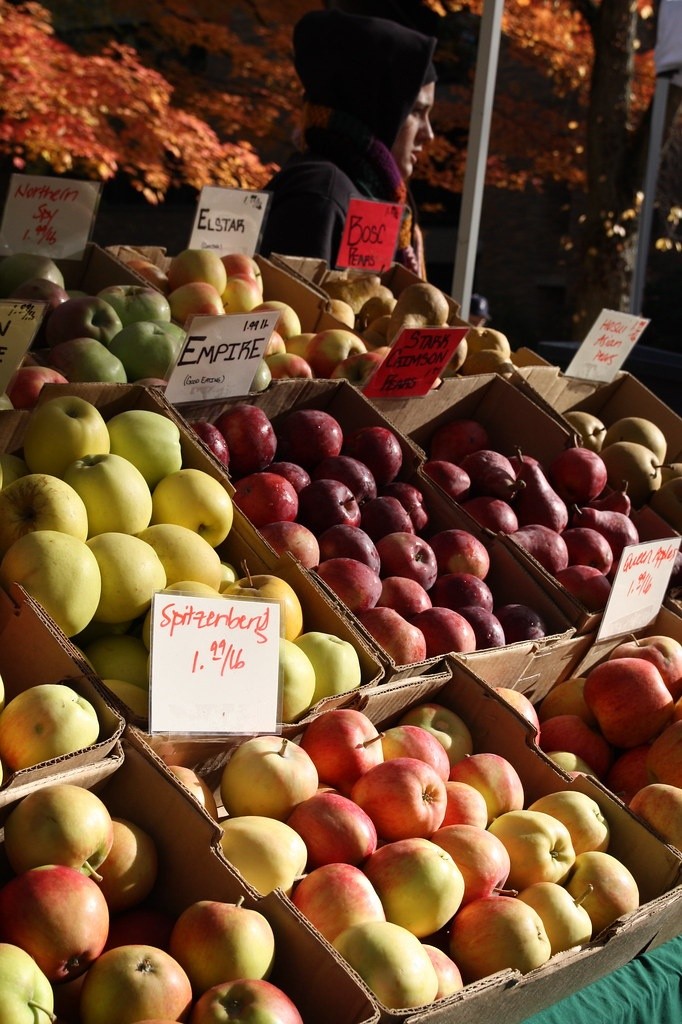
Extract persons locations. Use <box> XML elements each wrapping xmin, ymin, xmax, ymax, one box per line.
<box><xmin>260</xmin><ymin>11</ymin><xmax>439</xmax><ymax>280</ymax></box>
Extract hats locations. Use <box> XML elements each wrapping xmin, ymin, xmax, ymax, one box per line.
<box><xmin>423</xmin><ymin>62</ymin><xmax>438</xmax><ymax>86</ymax></box>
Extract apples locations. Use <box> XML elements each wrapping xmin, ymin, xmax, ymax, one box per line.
<box><xmin>0</xmin><ymin>631</ymin><xmax>682</xmax><ymax>1024</ymax></box>
<box><xmin>2</xmin><ymin>394</ymin><xmax>547</xmax><ymax>791</ymax></box>
<box><xmin>0</xmin><ymin>252</ymin><xmax>392</xmax><ymax>414</ymax></box>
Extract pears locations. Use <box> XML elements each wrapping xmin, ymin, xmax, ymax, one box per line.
<box><xmin>421</xmin><ymin>417</ymin><xmax>639</xmax><ymax>612</ymax></box>
<box><xmin>562</xmin><ymin>410</ymin><xmax>682</xmax><ymax>549</ymax></box>
<box><xmin>320</xmin><ymin>270</ymin><xmax>518</xmax><ymax>382</ymax></box>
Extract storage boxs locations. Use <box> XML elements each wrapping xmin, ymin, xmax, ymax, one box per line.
<box><xmin>0</xmin><ymin>240</ymin><xmax>682</xmax><ymax>1024</ymax></box>
